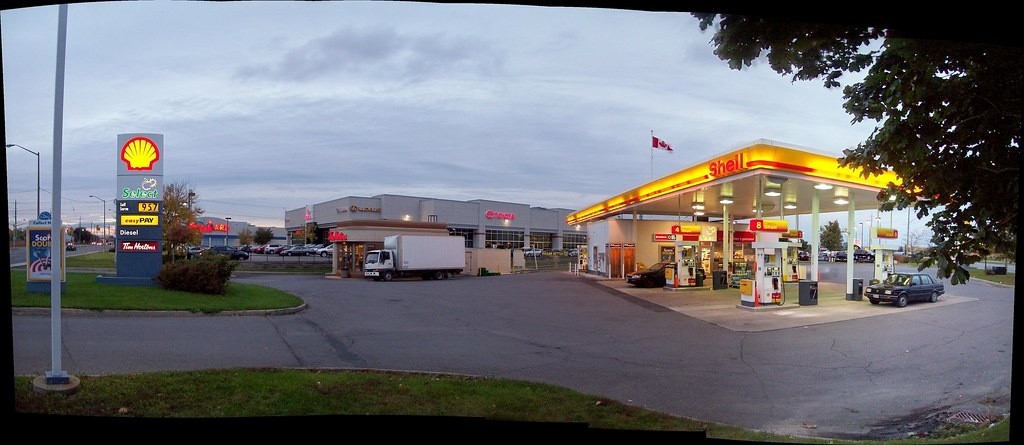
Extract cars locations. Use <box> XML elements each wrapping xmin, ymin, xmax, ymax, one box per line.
<box><xmin>315</xmin><ymin>243</ymin><xmax>347</xmax><ymax>258</ymax></box>
<box><xmin>523</xmin><ymin>247</ymin><xmax>542</xmax><ymax>257</ymax></box>
<box><xmin>188</xmin><ymin>243</ymin><xmax>327</xmax><ymax>255</ymax></box>
<box><xmin>540</xmin><ymin>246</ymin><xmax>579</xmax><ymax>258</ymax></box>
<box><xmin>798</xmin><ymin>250</ymin><xmax>811</xmax><ymax>261</ymax></box>
<box><xmin>819</xmin><ymin>245</ymin><xmax>875</xmax><ymax>264</ymax></box>
<box><xmin>66</xmin><ymin>245</ymin><xmax>76</xmax><ymax>252</ymax></box>
<box><xmin>280</xmin><ymin>245</ymin><xmax>316</xmax><ymax>257</ymax></box>
<box><xmin>865</xmin><ymin>272</ymin><xmax>946</xmax><ymax>308</ymax></box>
<box><xmin>91</xmin><ymin>240</ymin><xmax>115</xmax><ymax>251</ymax></box>
<box><xmin>208</xmin><ymin>245</ymin><xmax>249</xmax><ymax>263</ymax></box>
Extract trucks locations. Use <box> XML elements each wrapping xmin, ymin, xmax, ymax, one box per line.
<box><xmin>364</xmin><ymin>233</ymin><xmax>465</xmax><ymax>282</ymax></box>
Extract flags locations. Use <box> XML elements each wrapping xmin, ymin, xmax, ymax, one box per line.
<box><xmin>652</xmin><ymin>136</ymin><xmax>674</xmax><ymax>152</ymax></box>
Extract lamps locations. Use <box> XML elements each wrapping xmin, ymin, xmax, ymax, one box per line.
<box><xmin>783</xmin><ymin>201</ymin><xmax>797</xmax><ymax>210</ymax></box>
<box><xmin>691</xmin><ymin>200</ymin><xmax>705</xmax><ymax>210</ymax></box>
<box><xmin>719</xmin><ymin>196</ymin><xmax>734</xmax><ymax>204</ymax></box>
<box><xmin>753</xmin><ymin>205</ymin><xmax>763</xmax><ymax>212</ymax></box>
<box><xmin>764</xmin><ymin>187</ymin><xmax>781</xmax><ymax>196</ymax></box>
<box><xmin>833</xmin><ymin>196</ymin><xmax>850</xmax><ymax>206</ymax></box>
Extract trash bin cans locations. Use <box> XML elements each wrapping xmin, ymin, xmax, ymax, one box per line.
<box><xmin>853</xmin><ymin>278</ymin><xmax>864</xmax><ymax>301</ymax></box>
<box><xmin>341</xmin><ymin>268</ymin><xmax>349</xmax><ymax>278</ymax></box>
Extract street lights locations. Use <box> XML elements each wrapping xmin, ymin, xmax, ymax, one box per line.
<box><xmin>859</xmin><ymin>223</ymin><xmax>864</xmax><ymax>249</ymax></box>
<box><xmin>73</xmin><ymin>208</ymin><xmax>82</xmax><ymax>244</ymax></box>
<box><xmin>89</xmin><ymin>195</ymin><xmax>105</xmax><ymax>240</ymax></box>
<box><xmin>225</xmin><ymin>217</ymin><xmax>232</xmax><ymax>246</ymax></box>
<box><xmin>6</xmin><ymin>144</ymin><xmax>40</xmax><ymax>220</ymax></box>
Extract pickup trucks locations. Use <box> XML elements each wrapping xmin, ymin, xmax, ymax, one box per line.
<box><xmin>625</xmin><ymin>262</ymin><xmax>706</xmax><ymax>288</ymax></box>
